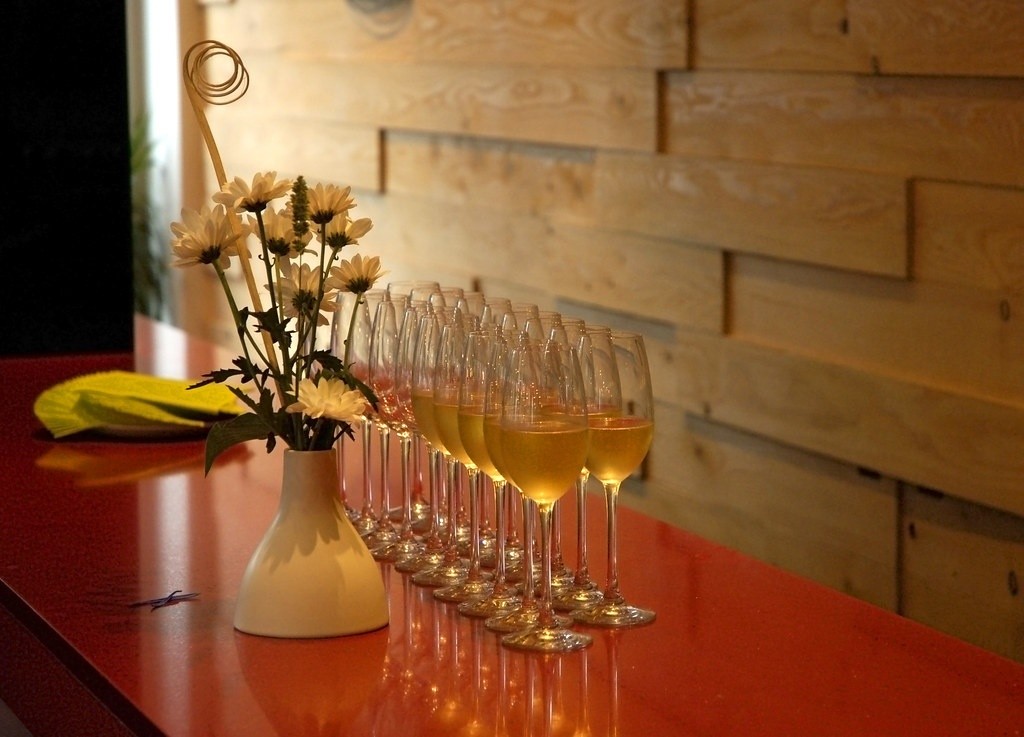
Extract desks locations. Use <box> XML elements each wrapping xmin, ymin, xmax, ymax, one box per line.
<box><xmin>0</xmin><ymin>309</ymin><xmax>1024</xmax><ymax>737</ymax></box>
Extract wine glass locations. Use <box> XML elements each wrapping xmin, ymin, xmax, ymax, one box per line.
<box><xmin>329</xmin><ymin>280</ymin><xmax>655</xmax><ymax>653</ymax></box>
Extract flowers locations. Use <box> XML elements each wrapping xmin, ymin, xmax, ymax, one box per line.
<box><xmin>170</xmin><ymin>39</ymin><xmax>391</xmax><ymax>479</ymax></box>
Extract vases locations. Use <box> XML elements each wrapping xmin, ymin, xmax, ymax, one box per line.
<box><xmin>233</xmin><ymin>449</ymin><xmax>386</xmax><ymax>637</ymax></box>
